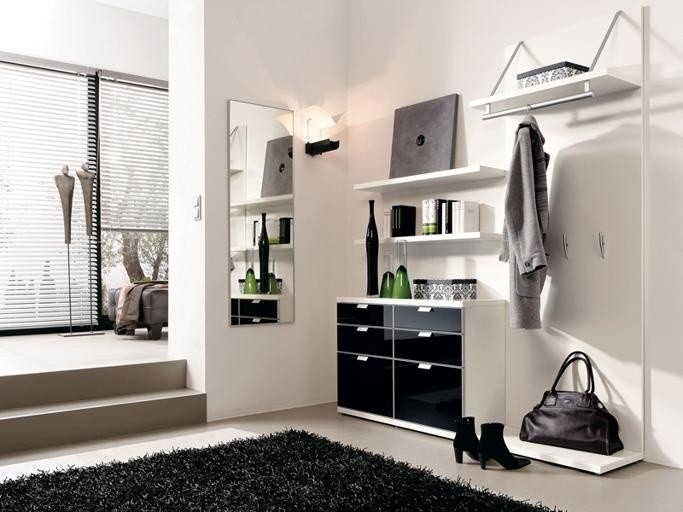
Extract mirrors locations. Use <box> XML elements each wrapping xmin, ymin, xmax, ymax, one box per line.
<box><xmin>224</xmin><ymin>98</ymin><xmax>297</xmax><ymax>328</ymax></box>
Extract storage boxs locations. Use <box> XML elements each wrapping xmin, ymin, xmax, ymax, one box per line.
<box><xmin>412</xmin><ymin>278</ymin><xmax>477</xmax><ymax>302</ymax></box>
<box><xmin>515</xmin><ymin>61</ymin><xmax>589</xmax><ymax>90</ymax></box>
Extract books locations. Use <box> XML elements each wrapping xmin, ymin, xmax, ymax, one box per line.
<box><xmin>380</xmin><ymin>198</ymin><xmax>480</xmax><ymax>238</ymax></box>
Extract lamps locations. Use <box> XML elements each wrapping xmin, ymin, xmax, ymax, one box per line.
<box><xmin>303</xmin><ymin>104</ymin><xmax>340</xmax><ymax>160</ymax></box>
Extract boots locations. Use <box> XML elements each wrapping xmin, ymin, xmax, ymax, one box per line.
<box><xmin>479</xmin><ymin>423</ymin><xmax>533</xmax><ymax>470</ymax></box>
<box><xmin>453</xmin><ymin>417</ymin><xmax>492</xmax><ymax>463</ymax></box>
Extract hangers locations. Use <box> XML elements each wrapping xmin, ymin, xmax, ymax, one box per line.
<box><xmin>525</xmin><ymin>104</ymin><xmax>532</xmax><ymax>113</ymax></box>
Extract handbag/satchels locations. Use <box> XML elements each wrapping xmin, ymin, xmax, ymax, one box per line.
<box><xmin>519</xmin><ymin>389</ymin><xmax>624</xmax><ymax>456</ymax></box>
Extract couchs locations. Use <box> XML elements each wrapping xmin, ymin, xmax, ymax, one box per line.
<box><xmin>106</xmin><ymin>283</ymin><xmax>168</xmax><ymax>341</ymax></box>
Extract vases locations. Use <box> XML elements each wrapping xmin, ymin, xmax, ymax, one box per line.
<box><xmin>258</xmin><ymin>212</ymin><xmax>269</xmax><ymax>294</ymax></box>
<box><xmin>365</xmin><ymin>199</ymin><xmax>379</xmax><ymax>296</ymax></box>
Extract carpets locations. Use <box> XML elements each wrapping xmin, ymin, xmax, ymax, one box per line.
<box><xmin>0</xmin><ymin>427</ymin><xmax>570</xmax><ymax>512</ymax></box>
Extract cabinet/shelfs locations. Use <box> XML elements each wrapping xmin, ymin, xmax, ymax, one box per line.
<box><xmin>350</xmin><ymin>7</ymin><xmax>642</xmax><ymax>250</ymax></box>
<box><xmin>330</xmin><ymin>294</ymin><xmax>509</xmax><ymax>441</ymax></box>
<box><xmin>226</xmin><ymin>193</ymin><xmax>294</xmax><ymax>253</ymax></box>
<box><xmin>228</xmin><ymin>291</ymin><xmax>294</xmax><ymax>327</ymax></box>
